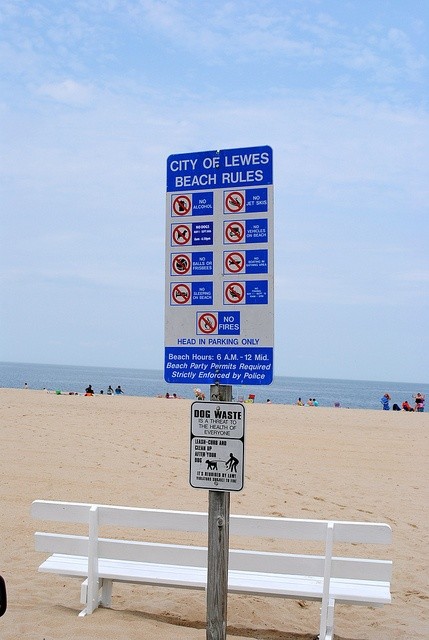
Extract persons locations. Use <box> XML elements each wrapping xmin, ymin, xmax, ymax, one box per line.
<box><xmin>380</xmin><ymin>393</ymin><xmax>391</xmax><ymax>410</ymax></box>
<box><xmin>412</xmin><ymin>393</ymin><xmax>424</xmax><ymax>411</ymax></box>
<box><xmin>296</xmin><ymin>398</ymin><xmax>302</xmax><ymax>404</ymax></box>
<box><xmin>174</xmin><ymin>393</ymin><xmax>176</xmax><ymax>397</ymax></box>
<box><xmin>402</xmin><ymin>400</ymin><xmax>413</xmax><ymax>411</ymax></box>
<box><xmin>166</xmin><ymin>393</ymin><xmax>168</xmax><ymax>396</ymax></box>
<box><xmin>107</xmin><ymin>386</ymin><xmax>113</xmax><ymax>395</ymax></box>
<box><xmin>115</xmin><ymin>386</ymin><xmax>123</xmax><ymax>394</ymax></box>
<box><xmin>24</xmin><ymin>382</ymin><xmax>28</xmax><ymax>388</ymax></box>
<box><xmin>306</xmin><ymin>399</ymin><xmax>312</xmax><ymax>406</ymax></box>
<box><xmin>267</xmin><ymin>399</ymin><xmax>270</xmax><ymax>402</ymax></box>
<box><xmin>313</xmin><ymin>399</ymin><xmax>318</xmax><ymax>406</ymax></box>
<box><xmin>85</xmin><ymin>384</ymin><xmax>94</xmax><ymax>392</ymax></box>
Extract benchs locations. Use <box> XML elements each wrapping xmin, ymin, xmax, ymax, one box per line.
<box><xmin>31</xmin><ymin>500</ymin><xmax>393</xmax><ymax>639</ymax></box>
